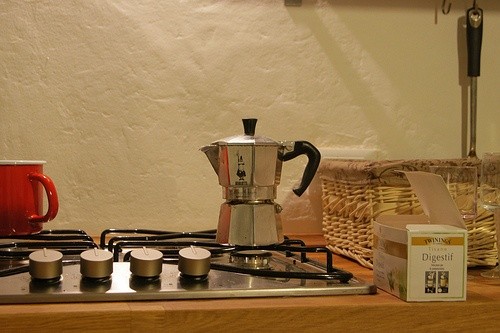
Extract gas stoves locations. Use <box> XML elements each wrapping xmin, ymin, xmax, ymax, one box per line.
<box><xmin>0</xmin><ymin>228</ymin><xmax>377</xmax><ymax>304</ymax></box>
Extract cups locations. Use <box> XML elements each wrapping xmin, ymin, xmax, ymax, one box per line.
<box><xmin>0</xmin><ymin>160</ymin><xmax>58</xmax><ymax>235</ymax></box>
<box><xmin>428</xmin><ymin>166</ymin><xmax>476</xmax><ymax>220</ymax></box>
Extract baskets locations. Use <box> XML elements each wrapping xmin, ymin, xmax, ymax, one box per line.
<box><xmin>318</xmin><ymin>154</ymin><xmax>500</xmax><ymax>267</ymax></box>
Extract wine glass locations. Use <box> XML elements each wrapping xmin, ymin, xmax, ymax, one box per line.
<box><xmin>479</xmin><ymin>152</ymin><xmax>500</xmax><ymax>278</ymax></box>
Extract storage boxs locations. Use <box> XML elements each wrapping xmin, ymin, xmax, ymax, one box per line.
<box><xmin>372</xmin><ymin>169</ymin><xmax>468</xmax><ymax>303</ymax></box>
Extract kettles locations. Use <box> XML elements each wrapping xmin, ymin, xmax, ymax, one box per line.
<box><xmin>198</xmin><ymin>118</ymin><xmax>321</xmax><ymax>246</ymax></box>
